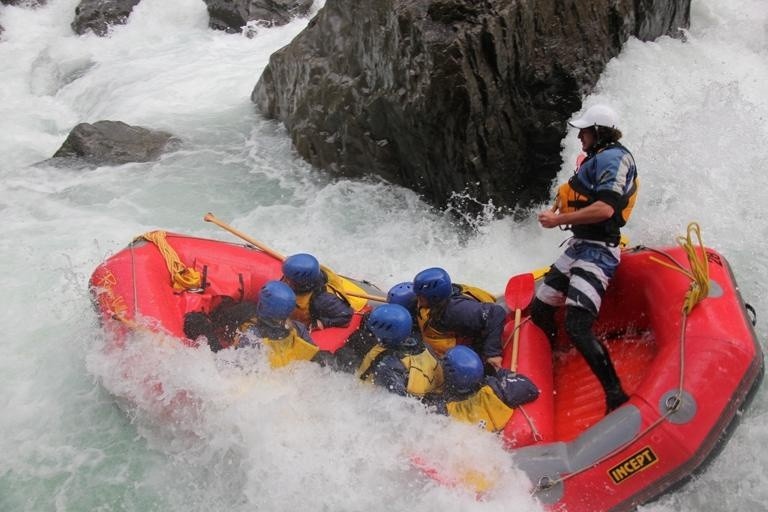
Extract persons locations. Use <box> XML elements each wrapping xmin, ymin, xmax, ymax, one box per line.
<box><xmin>387</xmin><ymin>278</ymin><xmax>418</xmax><ymax>326</ymax></box>
<box><xmin>311</xmin><ymin>305</ymin><xmax>442</xmax><ymax>400</ymax></box>
<box><xmin>422</xmin><ymin>344</ymin><xmax>539</xmax><ymax>431</ymax></box>
<box><xmin>414</xmin><ymin>268</ymin><xmax>504</xmax><ymax>370</ymax></box>
<box><xmin>278</xmin><ymin>253</ymin><xmax>370</xmax><ymax>331</ymax></box>
<box><xmin>534</xmin><ymin>103</ymin><xmax>635</xmax><ymax>416</ymax></box>
<box><xmin>185</xmin><ymin>283</ymin><xmax>317</xmax><ymax>367</ymax></box>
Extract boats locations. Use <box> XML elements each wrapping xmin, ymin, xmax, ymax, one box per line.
<box><xmin>88</xmin><ymin>229</ymin><xmax>765</xmax><ymax>511</ymax></box>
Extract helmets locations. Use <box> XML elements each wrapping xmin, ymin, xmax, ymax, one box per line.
<box><xmin>443</xmin><ymin>344</ymin><xmax>482</xmax><ymax>391</ymax></box>
<box><xmin>414</xmin><ymin>267</ymin><xmax>451</xmax><ymax>304</ymax></box>
<box><xmin>282</xmin><ymin>253</ymin><xmax>319</xmax><ymax>286</ymax></box>
<box><xmin>567</xmin><ymin>105</ymin><xmax>616</xmax><ymax>129</ymax></box>
<box><xmin>256</xmin><ymin>281</ymin><xmax>298</xmax><ymax>321</ymax></box>
<box><xmin>365</xmin><ymin>301</ymin><xmax>414</xmax><ymax>347</ymax></box>
<box><xmin>387</xmin><ymin>282</ymin><xmax>417</xmax><ymax>307</ymax></box>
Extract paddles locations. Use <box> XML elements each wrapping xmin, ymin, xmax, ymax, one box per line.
<box><xmin>204</xmin><ymin>212</ymin><xmax>368</xmax><ymax>312</ymax></box>
<box><xmin>504</xmin><ymin>273</ymin><xmax>535</xmax><ymax>372</ymax></box>
